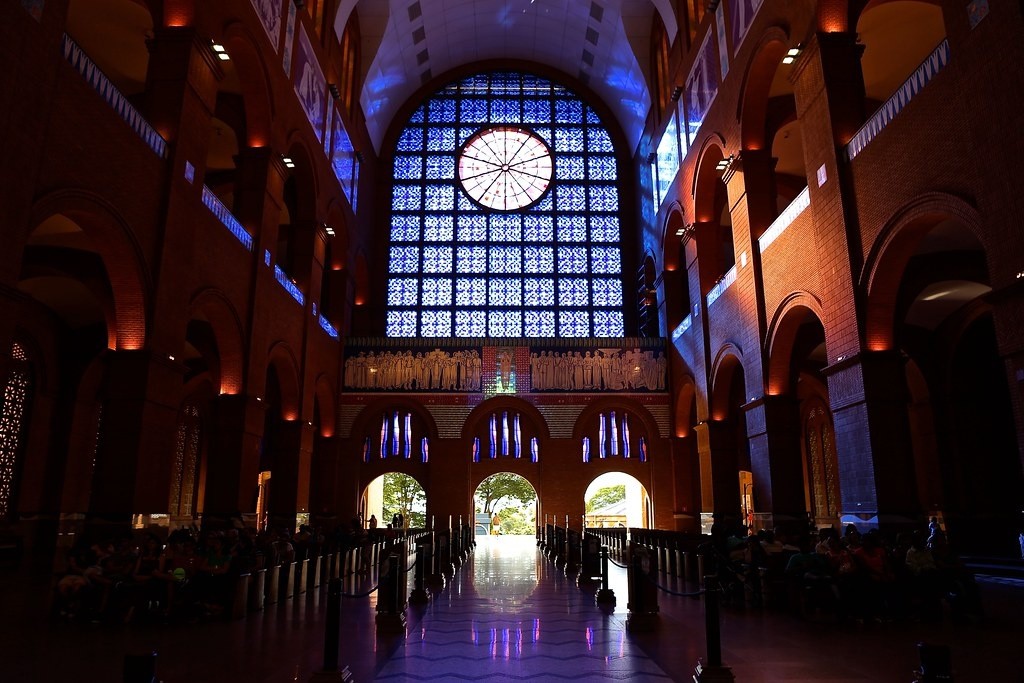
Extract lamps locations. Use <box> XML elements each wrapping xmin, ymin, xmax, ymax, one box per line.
<box><xmin>355</xmin><ymin>150</ymin><xmax>366</xmax><ymax>162</ymax></box>
<box><xmin>280</xmin><ymin>154</ymin><xmax>296</xmax><ymax>169</ymax></box>
<box><xmin>294</xmin><ymin>0</ymin><xmax>306</xmax><ymax>10</ymax></box>
<box><xmin>672</xmin><ymin>86</ymin><xmax>682</xmax><ymax>101</ymax></box>
<box><xmin>646</xmin><ymin>152</ymin><xmax>655</xmax><ymax>163</ymax></box>
<box><xmin>706</xmin><ymin>0</ymin><xmax>720</xmax><ymax>13</ymax></box>
<box><xmin>715</xmin><ymin>156</ymin><xmax>733</xmax><ymax>171</ymax></box>
<box><xmin>782</xmin><ymin>42</ymin><xmax>802</xmax><ymax>65</ymax></box>
<box><xmin>676</xmin><ymin>227</ymin><xmax>687</xmax><ymax>236</ymax></box>
<box><xmin>209</xmin><ymin>38</ymin><xmax>231</xmax><ymax>62</ymax></box>
<box><xmin>330</xmin><ymin>83</ymin><xmax>340</xmax><ymax>99</ymax></box>
<box><xmin>321</xmin><ymin>222</ymin><xmax>336</xmax><ymax>236</ymax></box>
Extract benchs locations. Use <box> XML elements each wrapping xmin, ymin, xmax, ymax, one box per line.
<box><xmin>586</xmin><ymin>526</ymin><xmax>952</xmax><ymax>628</ymax></box>
<box><xmin>47</xmin><ymin>529</ymin><xmax>429</xmax><ymax>638</ymax></box>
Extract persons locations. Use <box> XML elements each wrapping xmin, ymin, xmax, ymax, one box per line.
<box><xmin>227</xmin><ymin>511</ymin><xmax>244</xmax><ymax>528</ymax></box>
<box><xmin>365</xmin><ymin>515</ymin><xmax>378</xmax><ymax>529</ymax></box>
<box><xmin>727</xmin><ymin>525</ymin><xmax>934</xmax><ymax>577</ymax></box>
<box><xmin>926</xmin><ymin>516</ymin><xmax>945</xmax><ymax>548</ymax></box>
<box><xmin>56</xmin><ymin>525</ymin><xmax>325</xmax><ymax>632</ymax></box>
<box><xmin>384</xmin><ymin>524</ymin><xmax>396</xmax><ymax>540</ymax></box>
<box><xmin>391</xmin><ymin>514</ymin><xmax>403</xmax><ymax>528</ymax></box>
